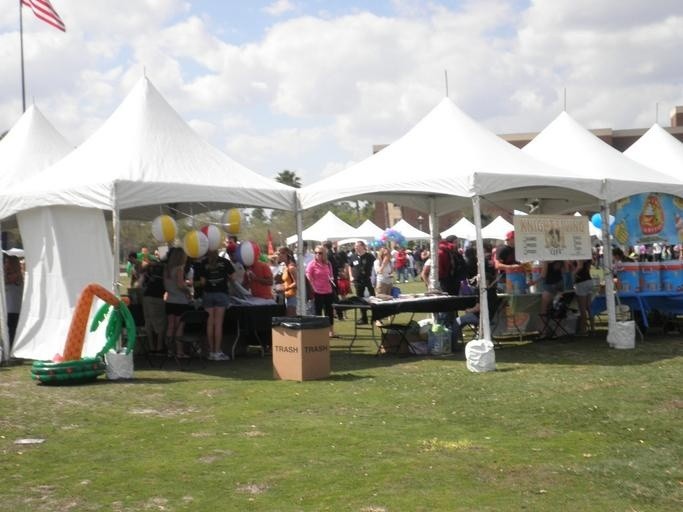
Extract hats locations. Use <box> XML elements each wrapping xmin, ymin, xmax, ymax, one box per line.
<box><xmin>504</xmin><ymin>231</ymin><xmax>514</xmax><ymax>241</ymax></box>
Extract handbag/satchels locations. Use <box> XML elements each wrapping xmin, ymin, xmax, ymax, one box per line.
<box><xmin>370</xmin><ymin>266</ymin><xmax>376</xmax><ymax>288</ymax></box>
<box><xmin>305</xmin><ymin>275</ymin><xmax>316</xmax><ymax>300</ymax></box>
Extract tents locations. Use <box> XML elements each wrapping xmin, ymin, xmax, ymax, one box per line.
<box><xmin>288</xmin><ymin>207</ymin><xmax>513</xmax><ymax>249</ymax></box>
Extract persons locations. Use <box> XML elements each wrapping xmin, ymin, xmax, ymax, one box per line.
<box><xmin>673</xmin><ymin>213</ymin><xmax>682</xmax><ymax>240</ymax></box>
<box><xmin>126</xmin><ymin>235</ymin><xmax>296</xmax><ymax>360</ymax></box>
<box><xmin>595</xmin><ymin>242</ymin><xmax>681</xmax><ymax>279</ymax></box>
<box><xmin>304</xmin><ymin>241</ymin><xmax>430</xmax><ymax>336</ymax></box>
<box><xmin>438</xmin><ymin>230</ymin><xmax>593</xmax><ymax>336</ymax></box>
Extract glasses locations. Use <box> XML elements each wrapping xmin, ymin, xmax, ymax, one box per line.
<box><xmin>314</xmin><ymin>252</ymin><xmax>322</xmax><ymax>254</ymax></box>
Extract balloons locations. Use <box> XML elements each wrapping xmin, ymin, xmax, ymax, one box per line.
<box><xmin>590</xmin><ymin>213</ymin><xmax>603</xmax><ymax>228</ymax></box>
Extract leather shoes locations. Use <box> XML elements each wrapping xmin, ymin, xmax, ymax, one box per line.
<box><xmin>534</xmin><ymin>335</ymin><xmax>550</xmax><ymax>341</ymax></box>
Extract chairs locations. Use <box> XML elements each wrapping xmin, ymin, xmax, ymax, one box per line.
<box><xmin>373</xmin><ymin>302</ymin><xmax>418</xmax><ymax>358</ymax></box>
<box><xmin>465</xmin><ymin>292</ymin><xmax>577</xmax><ymax>350</ymax></box>
<box><xmin>118</xmin><ymin>304</ymin><xmax>210</xmax><ymax>371</ymax></box>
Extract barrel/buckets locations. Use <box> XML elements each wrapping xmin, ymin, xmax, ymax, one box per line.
<box><xmin>662</xmin><ymin>259</ymin><xmax>682</xmax><ymax>292</ymax></box>
<box><xmin>505</xmin><ymin>264</ymin><xmax>528</xmax><ymax>294</ymax></box>
<box><xmin>617</xmin><ymin>260</ymin><xmax>639</xmax><ymax>294</ymax></box>
<box><xmin>640</xmin><ymin>260</ymin><xmax>661</xmax><ymax>293</ymax></box>
<box><xmin>429</xmin><ymin>327</ymin><xmax>453</xmax><ymax>355</ymax></box>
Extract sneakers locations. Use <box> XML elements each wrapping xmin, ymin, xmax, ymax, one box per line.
<box><xmin>207</xmin><ymin>352</ymin><xmax>230</xmax><ymax>360</ymax></box>
<box><xmin>167</xmin><ymin>351</ymin><xmax>192</xmax><ymax>358</ymax></box>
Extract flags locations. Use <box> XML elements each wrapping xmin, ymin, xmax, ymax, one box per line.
<box><xmin>20</xmin><ymin>0</ymin><xmax>66</xmax><ymax>33</ymax></box>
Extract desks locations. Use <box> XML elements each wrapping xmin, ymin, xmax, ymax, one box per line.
<box><xmin>595</xmin><ymin>292</ymin><xmax>683</xmax><ymax>342</ymax></box>
<box><xmin>330</xmin><ymin>294</ymin><xmax>542</xmax><ymax>356</ymax></box>
<box><xmin>225</xmin><ymin>303</ymin><xmax>282</xmax><ymax>360</ymax></box>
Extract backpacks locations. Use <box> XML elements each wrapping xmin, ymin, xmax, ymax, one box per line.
<box><xmin>438</xmin><ymin>246</ymin><xmax>467</xmax><ymax>279</ymax></box>
